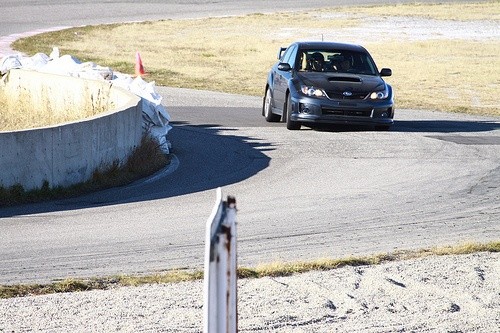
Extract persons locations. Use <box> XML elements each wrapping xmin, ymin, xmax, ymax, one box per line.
<box><xmin>336</xmin><ymin>58</ymin><xmax>352</xmax><ymax>73</ymax></box>
<box><xmin>301</xmin><ymin>52</ymin><xmax>331</xmax><ymax>73</ymax></box>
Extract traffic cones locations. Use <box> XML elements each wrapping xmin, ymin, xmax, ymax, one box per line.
<box><xmin>135</xmin><ymin>52</ymin><xmax>144</xmax><ymax>74</ymax></box>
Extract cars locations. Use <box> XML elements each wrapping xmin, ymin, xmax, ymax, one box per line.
<box><xmin>262</xmin><ymin>41</ymin><xmax>395</xmax><ymax>130</ymax></box>
